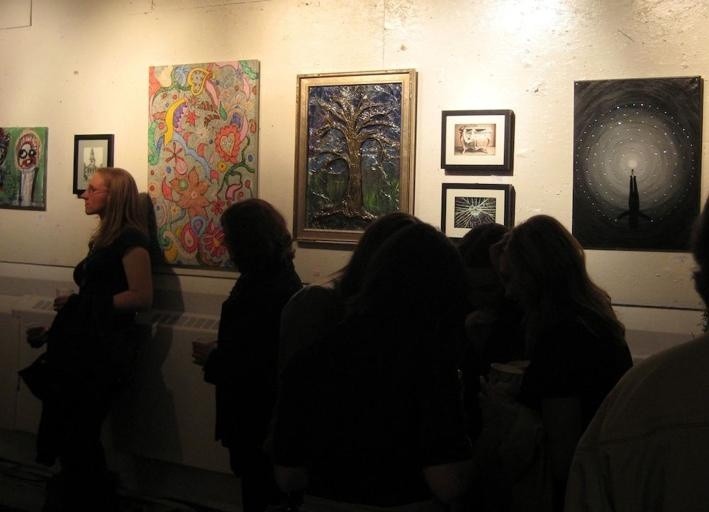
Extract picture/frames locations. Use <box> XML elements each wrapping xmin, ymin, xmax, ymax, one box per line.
<box><xmin>292</xmin><ymin>68</ymin><xmax>515</xmax><ymax>244</ymax></box>
<box><xmin>72</xmin><ymin>134</ymin><xmax>115</xmax><ymax>195</ymax></box>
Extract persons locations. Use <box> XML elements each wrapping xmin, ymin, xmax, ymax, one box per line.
<box><xmin>270</xmin><ymin>194</ymin><xmax>709</xmax><ymax>512</ymax></box>
<box><xmin>15</xmin><ymin>166</ymin><xmax>156</xmax><ymax>511</ymax></box>
<box><xmin>190</xmin><ymin>198</ymin><xmax>304</xmax><ymax>510</ymax></box>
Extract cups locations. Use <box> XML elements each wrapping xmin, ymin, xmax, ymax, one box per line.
<box><xmin>192</xmin><ymin>336</ymin><xmax>217</xmax><ymax>364</ymax></box>
<box><xmin>490</xmin><ymin>362</ymin><xmax>524</xmax><ymax>398</ymax></box>
<box><xmin>56</xmin><ymin>286</ymin><xmax>73</xmax><ymax>310</ymax></box>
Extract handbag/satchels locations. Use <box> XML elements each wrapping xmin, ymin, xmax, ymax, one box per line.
<box><xmin>16</xmin><ymin>350</ymin><xmax>144</xmax><ymax>411</ymax></box>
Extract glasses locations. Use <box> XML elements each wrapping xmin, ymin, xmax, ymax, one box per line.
<box><xmin>87</xmin><ymin>184</ymin><xmax>110</xmax><ymax>194</ymax></box>
